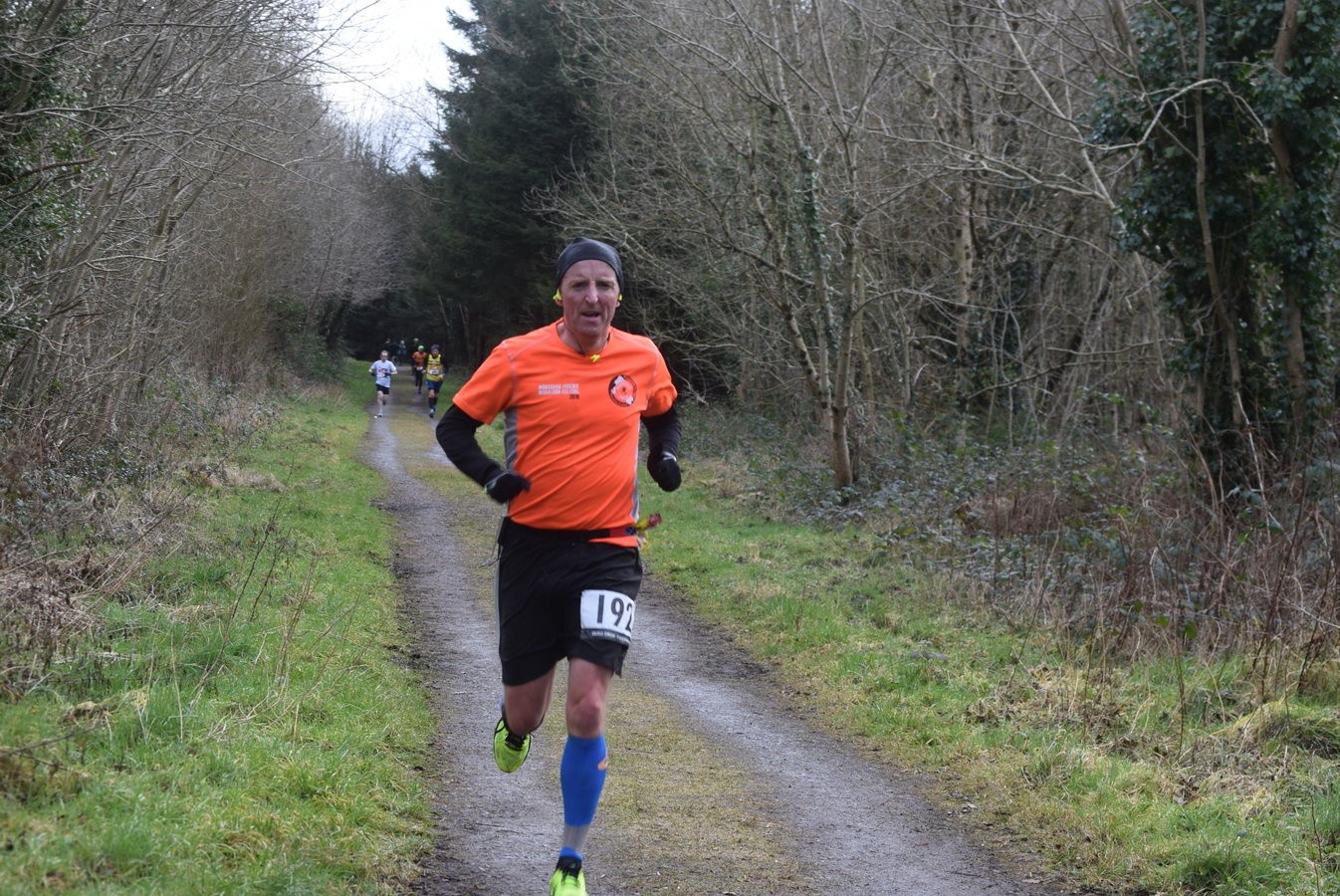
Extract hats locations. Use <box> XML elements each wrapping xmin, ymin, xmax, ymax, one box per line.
<box><xmin>554</xmin><ymin>236</ymin><xmax>625</xmax><ymax>294</ymax></box>
<box><xmin>414</xmin><ymin>337</ymin><xmax>440</xmax><ymax>352</ymax></box>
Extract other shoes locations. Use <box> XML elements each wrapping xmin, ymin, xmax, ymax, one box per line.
<box><xmin>377</xmin><ymin>383</ymin><xmax>435</xmax><ymax>417</ymax></box>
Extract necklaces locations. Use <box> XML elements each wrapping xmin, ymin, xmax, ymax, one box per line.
<box><xmin>563</xmin><ymin>321</ymin><xmax>610</xmax><ymax>363</ymax></box>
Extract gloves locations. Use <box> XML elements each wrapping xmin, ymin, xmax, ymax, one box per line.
<box><xmin>485</xmin><ymin>468</ymin><xmax>530</xmax><ymax>503</ymax></box>
<box><xmin>646</xmin><ymin>452</ymin><xmax>681</xmax><ymax>492</ymax></box>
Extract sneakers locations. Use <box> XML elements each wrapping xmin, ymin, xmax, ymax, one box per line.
<box><xmin>493</xmin><ymin>716</ymin><xmax>533</xmax><ymax>774</ymax></box>
<box><xmin>548</xmin><ymin>868</ymin><xmax>586</xmax><ymax>895</ymax></box>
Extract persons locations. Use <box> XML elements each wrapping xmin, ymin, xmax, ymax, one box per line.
<box><xmin>412</xmin><ymin>344</ymin><xmax>427</xmax><ymax>394</ymax></box>
<box><xmin>433</xmin><ymin>234</ymin><xmax>682</xmax><ymax>896</ymax></box>
<box><xmin>369</xmin><ymin>350</ymin><xmax>398</xmax><ymax>419</ymax></box>
<box><xmin>410</xmin><ymin>339</ymin><xmax>422</xmax><ymax>377</ymax></box>
<box><xmin>423</xmin><ymin>344</ymin><xmax>449</xmax><ymax>418</ymax></box>
<box><xmin>396</xmin><ymin>341</ymin><xmax>407</xmax><ymax>365</ymax></box>
